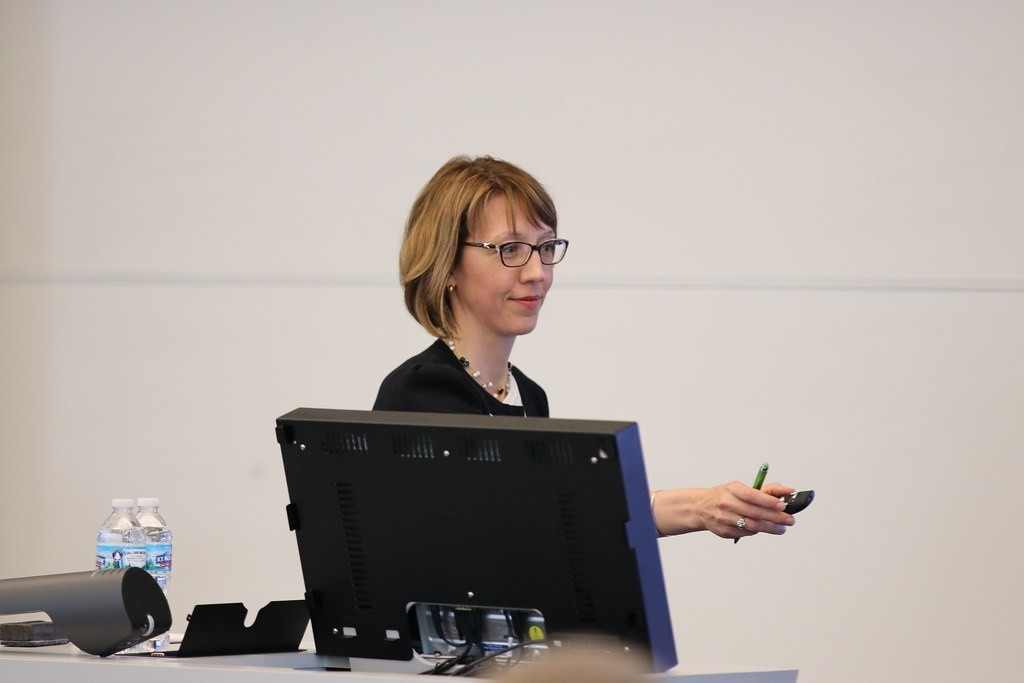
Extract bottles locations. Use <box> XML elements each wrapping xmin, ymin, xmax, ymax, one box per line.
<box><xmin>95</xmin><ymin>499</ymin><xmax>173</xmax><ymax>654</ymax></box>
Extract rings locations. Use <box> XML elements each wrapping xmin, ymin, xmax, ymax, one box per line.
<box><xmin>737</xmin><ymin>516</ymin><xmax>746</xmax><ymax>531</ymax></box>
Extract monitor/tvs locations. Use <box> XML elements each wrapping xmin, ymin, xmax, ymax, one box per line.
<box><xmin>277</xmin><ymin>406</ymin><xmax>680</xmax><ymax>672</ymax></box>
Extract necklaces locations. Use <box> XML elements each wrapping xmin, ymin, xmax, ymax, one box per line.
<box><xmin>447</xmin><ymin>341</ymin><xmax>512</xmax><ymax>399</ymax></box>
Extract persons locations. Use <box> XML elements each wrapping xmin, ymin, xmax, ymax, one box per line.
<box><xmin>368</xmin><ymin>152</ymin><xmax>795</xmax><ymax>538</ymax></box>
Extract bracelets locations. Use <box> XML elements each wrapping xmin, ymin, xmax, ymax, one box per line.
<box><xmin>650</xmin><ymin>489</ymin><xmax>673</xmax><ymax>538</ymax></box>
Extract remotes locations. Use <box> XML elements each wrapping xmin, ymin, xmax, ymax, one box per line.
<box><xmin>772</xmin><ymin>483</ymin><xmax>815</xmax><ymax>515</ymax></box>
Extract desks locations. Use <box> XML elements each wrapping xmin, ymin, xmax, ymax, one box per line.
<box><xmin>0</xmin><ymin>613</ymin><xmax>802</xmax><ymax>682</ymax></box>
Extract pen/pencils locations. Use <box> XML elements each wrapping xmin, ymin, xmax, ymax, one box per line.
<box><xmin>733</xmin><ymin>462</ymin><xmax>771</xmax><ymax>545</ymax></box>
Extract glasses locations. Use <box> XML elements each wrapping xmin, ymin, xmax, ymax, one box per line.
<box><xmin>463</xmin><ymin>238</ymin><xmax>569</xmax><ymax>267</ymax></box>
